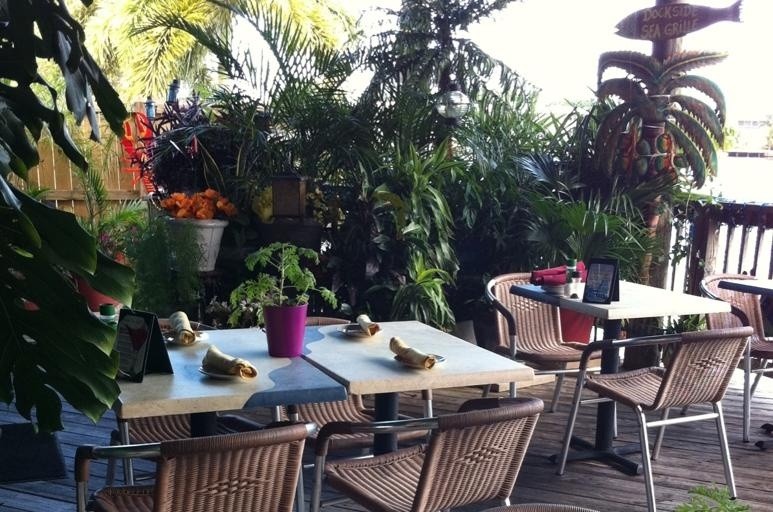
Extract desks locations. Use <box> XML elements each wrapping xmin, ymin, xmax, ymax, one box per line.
<box><xmin>111</xmin><ymin>326</ymin><xmax>349</xmax><ymax>487</ymax></box>
<box><xmin>290</xmin><ymin>318</ymin><xmax>533</xmax><ymax>452</ymax></box>
<box><xmin>717</xmin><ymin>279</ymin><xmax>773</xmax><ymax>296</ymax></box>
<box><xmin>510</xmin><ymin>271</ymin><xmax>731</xmax><ymax>476</ymax></box>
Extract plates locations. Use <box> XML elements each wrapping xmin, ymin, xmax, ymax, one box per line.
<box><xmin>198</xmin><ymin>367</ymin><xmax>235</xmax><ymax>380</ymax></box>
<box><xmin>167</xmin><ymin>331</ymin><xmax>208</xmax><ymax>345</ymax></box>
<box><xmin>394</xmin><ymin>352</ymin><xmax>446</xmax><ymax>370</ymax></box>
<box><xmin>336</xmin><ymin>324</ymin><xmax>384</xmax><ymax>338</ymax></box>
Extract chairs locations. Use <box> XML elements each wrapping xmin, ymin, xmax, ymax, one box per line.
<box><xmin>701</xmin><ymin>273</ymin><xmax>773</xmax><ymax>442</ymax></box>
<box><xmin>105</xmin><ymin>311</ymin><xmax>265</xmax><ymax>483</ymax></box>
<box><xmin>488</xmin><ymin>272</ymin><xmax>605</xmax><ymax>398</ymax></box>
<box><xmin>553</xmin><ymin>327</ymin><xmax>753</xmax><ymax>502</ymax></box>
<box><xmin>69</xmin><ymin>420</ymin><xmax>316</xmax><ymax>512</ymax></box>
<box><xmin>273</xmin><ymin>311</ymin><xmax>430</xmax><ymax>511</ymax></box>
<box><xmin>315</xmin><ymin>397</ymin><xmax>545</xmax><ymax>511</ymax></box>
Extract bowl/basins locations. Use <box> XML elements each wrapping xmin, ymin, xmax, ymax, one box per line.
<box><xmin>540</xmin><ymin>285</ymin><xmax>564</xmax><ymax>295</ymax></box>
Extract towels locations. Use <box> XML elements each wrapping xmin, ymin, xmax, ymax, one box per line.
<box><xmin>201</xmin><ymin>345</ymin><xmax>260</xmax><ymax>381</ymax></box>
<box><xmin>391</xmin><ymin>336</ymin><xmax>439</xmax><ymax>367</ymax></box>
<box><xmin>357</xmin><ymin>312</ymin><xmax>382</xmax><ymax>336</ymax></box>
<box><xmin>166</xmin><ymin>310</ymin><xmax>196</xmax><ymax>346</ymax></box>
<box><xmin>531</xmin><ymin>262</ymin><xmax>587</xmax><ymax>285</ymax></box>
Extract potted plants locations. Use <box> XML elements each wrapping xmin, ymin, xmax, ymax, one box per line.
<box><xmin>222</xmin><ymin>237</ymin><xmax>338</xmax><ymax>356</ymax></box>
<box><xmin>208</xmin><ymin>46</ymin><xmax>382</xmax><ymax>216</ymax></box>
<box><xmin>518</xmin><ymin>152</ymin><xmax>681</xmax><ymax>342</ymax></box>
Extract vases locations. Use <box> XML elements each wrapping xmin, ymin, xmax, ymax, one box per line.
<box><xmin>166</xmin><ymin>221</ymin><xmax>230</xmax><ymax>274</ymax></box>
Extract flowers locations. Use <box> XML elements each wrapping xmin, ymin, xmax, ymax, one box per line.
<box><xmin>155</xmin><ymin>186</ymin><xmax>237</xmax><ymax>219</ymax></box>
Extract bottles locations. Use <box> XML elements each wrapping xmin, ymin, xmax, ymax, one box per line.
<box><xmin>564</xmin><ymin>258</ymin><xmax>585</xmax><ymax>302</ymax></box>
